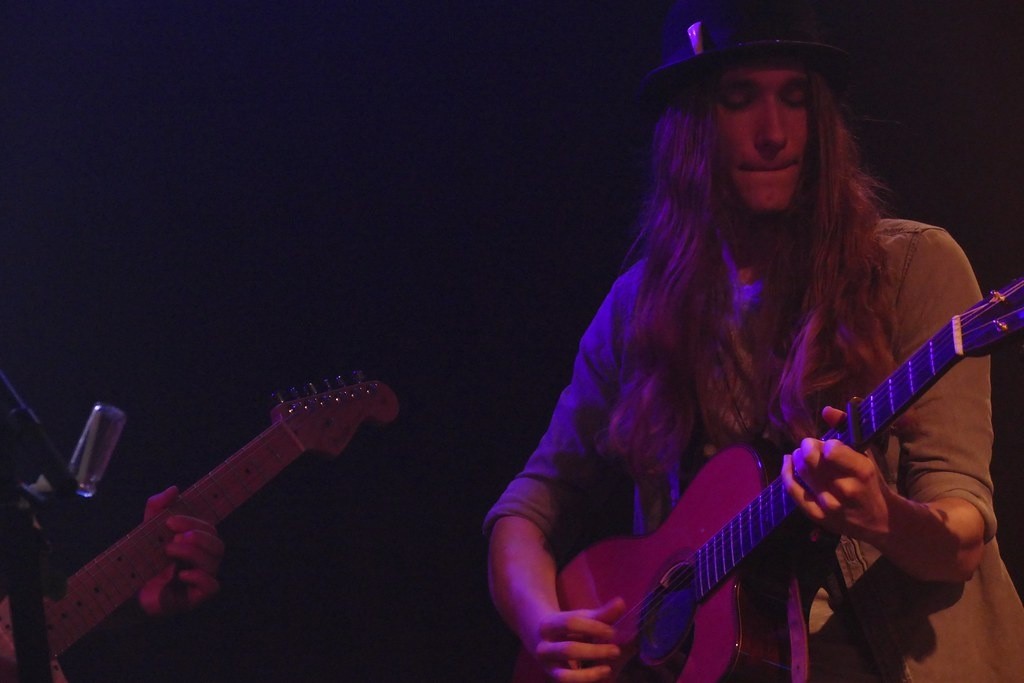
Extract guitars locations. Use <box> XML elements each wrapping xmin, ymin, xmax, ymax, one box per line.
<box><xmin>1</xmin><ymin>371</ymin><xmax>400</xmax><ymax>683</ymax></box>
<box><xmin>514</xmin><ymin>279</ymin><xmax>1024</xmax><ymax>683</ymax></box>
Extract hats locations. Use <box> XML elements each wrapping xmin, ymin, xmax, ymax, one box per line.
<box><xmin>639</xmin><ymin>0</ymin><xmax>847</xmax><ymax>108</ymax></box>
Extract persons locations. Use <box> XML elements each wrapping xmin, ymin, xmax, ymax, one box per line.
<box><xmin>481</xmin><ymin>0</ymin><xmax>1024</xmax><ymax>682</ymax></box>
